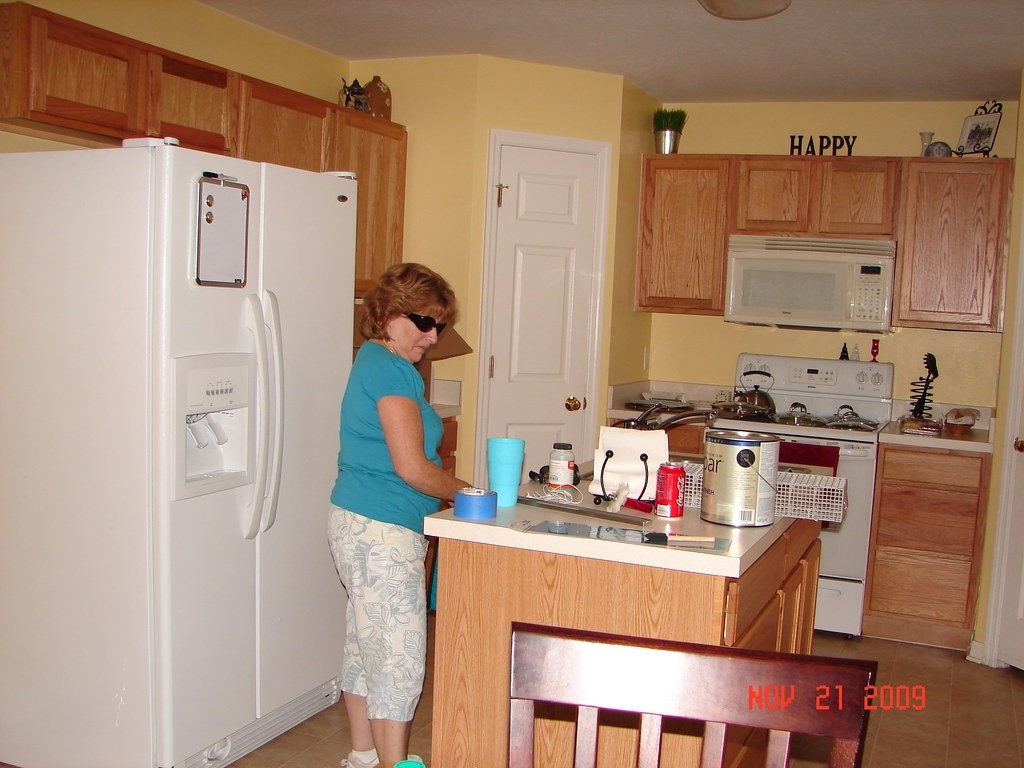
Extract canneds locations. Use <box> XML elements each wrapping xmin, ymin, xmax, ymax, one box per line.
<box><xmin>654</xmin><ymin>462</ymin><xmax>686</xmax><ymax>519</ymax></box>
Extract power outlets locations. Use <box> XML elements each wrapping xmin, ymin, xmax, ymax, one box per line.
<box><xmin>643</xmin><ymin>347</ymin><xmax>649</xmax><ymax>371</ymax></box>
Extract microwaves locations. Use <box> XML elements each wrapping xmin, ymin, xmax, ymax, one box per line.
<box><xmin>723</xmin><ymin>235</ymin><xmax>896</xmax><ymax>331</ymax></box>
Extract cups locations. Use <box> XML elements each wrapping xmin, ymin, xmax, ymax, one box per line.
<box><xmin>488</xmin><ymin>437</ymin><xmax>525</xmax><ymax>506</ymax></box>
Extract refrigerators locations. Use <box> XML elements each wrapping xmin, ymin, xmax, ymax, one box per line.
<box><xmin>0</xmin><ymin>135</ymin><xmax>359</xmax><ymax>768</ymax></box>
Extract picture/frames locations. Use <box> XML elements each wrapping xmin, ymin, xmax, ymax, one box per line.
<box><xmin>948</xmin><ymin>100</ymin><xmax>1007</xmax><ymax>158</ymax></box>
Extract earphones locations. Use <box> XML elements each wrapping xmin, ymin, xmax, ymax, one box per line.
<box><xmin>525</xmin><ymin>492</ymin><xmax>534</xmax><ymax>498</ymax></box>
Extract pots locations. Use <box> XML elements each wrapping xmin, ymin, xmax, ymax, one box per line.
<box><xmin>712</xmin><ymin>391</ymin><xmax>879</xmax><ymax>433</ymax></box>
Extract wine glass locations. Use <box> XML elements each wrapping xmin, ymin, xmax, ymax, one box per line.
<box><xmin>868</xmin><ymin>339</ymin><xmax>880</xmax><ymax>362</ymax></box>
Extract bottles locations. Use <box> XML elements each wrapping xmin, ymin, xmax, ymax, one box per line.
<box><xmin>547</xmin><ymin>442</ymin><xmax>574</xmax><ymax>494</ymax></box>
<box><xmin>363</xmin><ymin>75</ymin><xmax>392</xmax><ymax>121</ymax></box>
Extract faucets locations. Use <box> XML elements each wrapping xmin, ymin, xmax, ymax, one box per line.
<box><xmin>634</xmin><ymin>401</ymin><xmax>716</xmax><ymax>429</ymax></box>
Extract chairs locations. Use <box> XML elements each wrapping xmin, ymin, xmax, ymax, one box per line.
<box><xmin>504</xmin><ymin>618</ymin><xmax>879</xmax><ymax>768</ymax></box>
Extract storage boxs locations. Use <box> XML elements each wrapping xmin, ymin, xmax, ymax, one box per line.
<box><xmin>353</xmin><ymin>305</ymin><xmax>474</xmax><ymax>403</ymax></box>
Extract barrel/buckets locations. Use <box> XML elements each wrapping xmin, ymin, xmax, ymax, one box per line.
<box><xmin>700</xmin><ymin>430</ymin><xmax>780</xmax><ymax>526</ymax></box>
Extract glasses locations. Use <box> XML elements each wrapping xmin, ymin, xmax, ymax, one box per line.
<box><xmin>404</xmin><ymin>312</ymin><xmax>447</xmax><ymax>336</ymax></box>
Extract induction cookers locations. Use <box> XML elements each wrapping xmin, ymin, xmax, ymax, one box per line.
<box><xmin>709</xmin><ymin>353</ymin><xmax>894</xmax><ymax>442</ymax></box>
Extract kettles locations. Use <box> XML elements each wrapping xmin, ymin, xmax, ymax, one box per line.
<box><xmin>735</xmin><ymin>370</ymin><xmax>777</xmax><ymax>414</ymax></box>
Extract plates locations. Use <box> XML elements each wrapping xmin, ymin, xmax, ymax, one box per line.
<box><xmin>924</xmin><ymin>141</ymin><xmax>952</xmax><ymax>158</ymax></box>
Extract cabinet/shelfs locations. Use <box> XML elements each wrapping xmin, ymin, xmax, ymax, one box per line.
<box><xmin>630</xmin><ymin>154</ymin><xmax>730</xmax><ymax>313</ymax></box>
<box><xmin>728</xmin><ymin>153</ymin><xmax>899</xmax><ymax>240</ymax></box>
<box><xmin>862</xmin><ymin>444</ymin><xmax>998</xmax><ymax>653</ymax></box>
<box><xmin>894</xmin><ymin>157</ymin><xmax>1019</xmax><ymax>335</ymax></box>
<box><xmin>429</xmin><ymin>517</ymin><xmax>833</xmax><ymax>767</ymax></box>
<box><xmin>237</xmin><ymin>71</ymin><xmax>407</xmax><ymax>295</ymax></box>
<box><xmin>0</xmin><ymin>0</ymin><xmax>236</xmax><ymax>146</ymax></box>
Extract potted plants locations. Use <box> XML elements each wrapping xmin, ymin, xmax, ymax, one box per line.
<box><xmin>653</xmin><ymin>106</ymin><xmax>690</xmax><ymax>154</ymax></box>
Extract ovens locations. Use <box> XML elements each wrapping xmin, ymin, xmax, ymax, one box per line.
<box><xmin>703</xmin><ymin>426</ymin><xmax>878</xmax><ymax>639</ymax></box>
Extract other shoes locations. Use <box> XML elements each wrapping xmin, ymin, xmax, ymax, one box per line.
<box><xmin>341</xmin><ymin>752</ymin><xmax>423</xmax><ymax>768</ymax></box>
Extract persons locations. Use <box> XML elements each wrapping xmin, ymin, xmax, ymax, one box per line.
<box><xmin>326</xmin><ymin>261</ymin><xmax>473</xmax><ymax>768</ymax></box>
